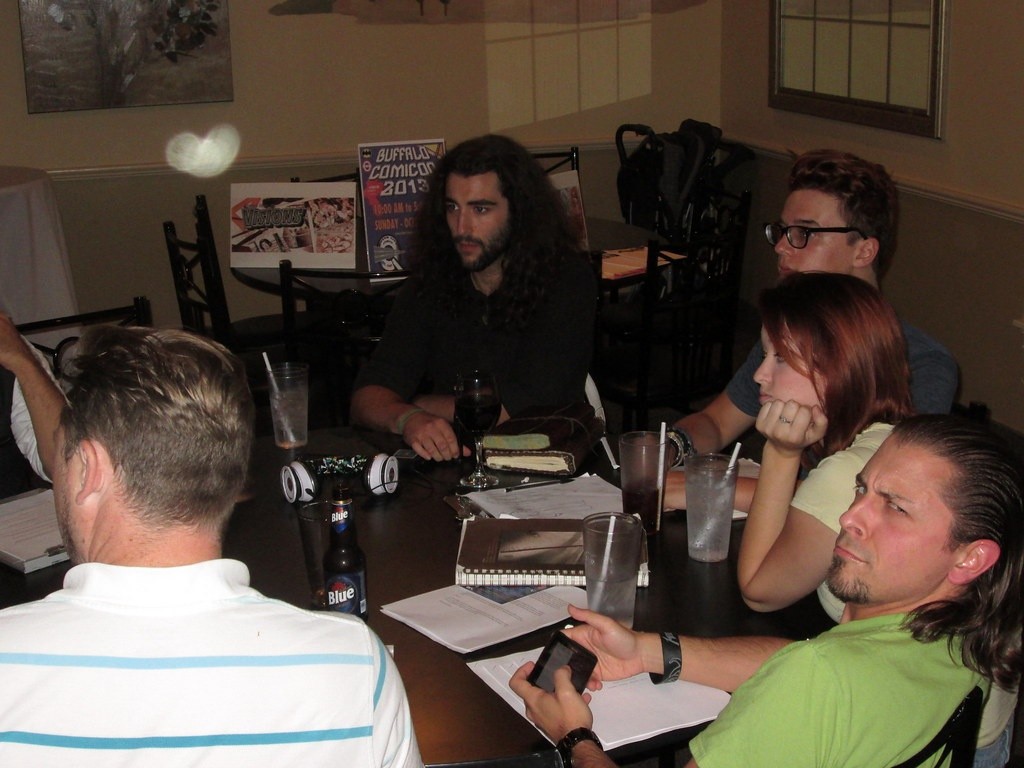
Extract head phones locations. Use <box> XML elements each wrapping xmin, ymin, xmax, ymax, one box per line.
<box><xmin>282</xmin><ymin>453</ymin><xmax>399</xmax><ymax>503</ymax></box>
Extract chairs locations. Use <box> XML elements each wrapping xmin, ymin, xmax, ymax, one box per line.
<box><xmin>529</xmin><ymin>144</ymin><xmax>751</xmax><ymax>436</ymax></box>
<box><xmin>161</xmin><ymin>219</ymin><xmax>330</xmax><ymax>427</ymax></box>
<box><xmin>279</xmin><ymin>259</ymin><xmax>434</xmax><ymax>419</ymax></box>
<box><xmin>0</xmin><ymin>296</ymin><xmax>154</xmax><ymax>474</ymax></box>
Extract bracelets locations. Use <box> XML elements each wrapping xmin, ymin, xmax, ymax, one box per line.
<box><xmin>399</xmin><ymin>408</ymin><xmax>425</xmax><ymax>432</ymax></box>
<box><xmin>666</xmin><ymin>425</ymin><xmax>697</xmax><ymax>467</ymax></box>
<box><xmin>649</xmin><ymin>631</ymin><xmax>682</xmax><ymax>684</ymax></box>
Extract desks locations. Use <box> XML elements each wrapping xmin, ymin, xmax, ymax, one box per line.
<box><xmin>0</xmin><ymin>165</ymin><xmax>82</xmax><ymax>326</ymax></box>
<box><xmin>229</xmin><ymin>213</ymin><xmax>669</xmax><ymax>388</ymax></box>
<box><xmin>0</xmin><ymin>426</ymin><xmax>834</xmax><ymax>768</ymax></box>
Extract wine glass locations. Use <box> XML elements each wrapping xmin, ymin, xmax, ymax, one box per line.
<box><xmin>454</xmin><ymin>369</ymin><xmax>502</xmax><ymax>487</ymax></box>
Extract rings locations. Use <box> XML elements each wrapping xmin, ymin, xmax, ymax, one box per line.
<box><xmin>780</xmin><ymin>416</ymin><xmax>793</xmax><ymax>425</ymax></box>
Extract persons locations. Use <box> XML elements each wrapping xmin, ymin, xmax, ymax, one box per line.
<box><xmin>635</xmin><ymin>148</ymin><xmax>959</xmax><ymax>514</ymax></box>
<box><xmin>508</xmin><ymin>413</ymin><xmax>1024</xmax><ymax>768</ymax></box>
<box><xmin>0</xmin><ymin>322</ymin><xmax>420</xmax><ymax>768</ymax></box>
<box><xmin>0</xmin><ymin>311</ymin><xmax>72</xmax><ymax>499</ymax></box>
<box><xmin>737</xmin><ymin>270</ymin><xmax>1019</xmax><ymax>768</ymax></box>
<box><xmin>350</xmin><ymin>134</ymin><xmax>596</xmax><ymax>462</ymax></box>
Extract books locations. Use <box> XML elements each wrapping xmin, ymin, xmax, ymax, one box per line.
<box><xmin>455</xmin><ymin>513</ymin><xmax>650</xmax><ymax>588</ymax></box>
<box><xmin>482</xmin><ymin>406</ymin><xmax>605</xmax><ymax>476</ymax></box>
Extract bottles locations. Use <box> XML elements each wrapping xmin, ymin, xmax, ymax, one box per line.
<box><xmin>325</xmin><ymin>483</ymin><xmax>369</xmax><ymax>624</ymax></box>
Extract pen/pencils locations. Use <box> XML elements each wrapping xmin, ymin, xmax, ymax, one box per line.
<box><xmin>505</xmin><ymin>480</ymin><xmax>575</xmax><ymax>493</ymax></box>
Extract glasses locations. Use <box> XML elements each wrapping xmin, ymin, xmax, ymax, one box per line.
<box><xmin>763</xmin><ymin>222</ymin><xmax>868</xmax><ymax>249</ymax></box>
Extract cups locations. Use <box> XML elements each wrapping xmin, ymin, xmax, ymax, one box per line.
<box><xmin>268</xmin><ymin>363</ymin><xmax>308</xmax><ymax>448</ymax></box>
<box><xmin>619</xmin><ymin>431</ymin><xmax>668</xmax><ymax>534</ymax></box>
<box><xmin>584</xmin><ymin>512</ymin><xmax>643</xmax><ymax>630</ymax></box>
<box><xmin>685</xmin><ymin>453</ymin><xmax>738</xmax><ymax>561</ymax></box>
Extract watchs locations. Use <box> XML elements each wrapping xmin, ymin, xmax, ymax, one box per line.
<box><xmin>553</xmin><ymin>727</ymin><xmax>603</xmax><ymax>768</ymax></box>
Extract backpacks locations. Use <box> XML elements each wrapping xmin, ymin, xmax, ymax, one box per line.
<box><xmin>615</xmin><ymin>118</ymin><xmax>741</xmax><ymax>303</ymax></box>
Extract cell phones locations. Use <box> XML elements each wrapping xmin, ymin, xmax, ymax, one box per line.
<box><xmin>527</xmin><ymin>631</ymin><xmax>598</xmax><ymax>696</ymax></box>
